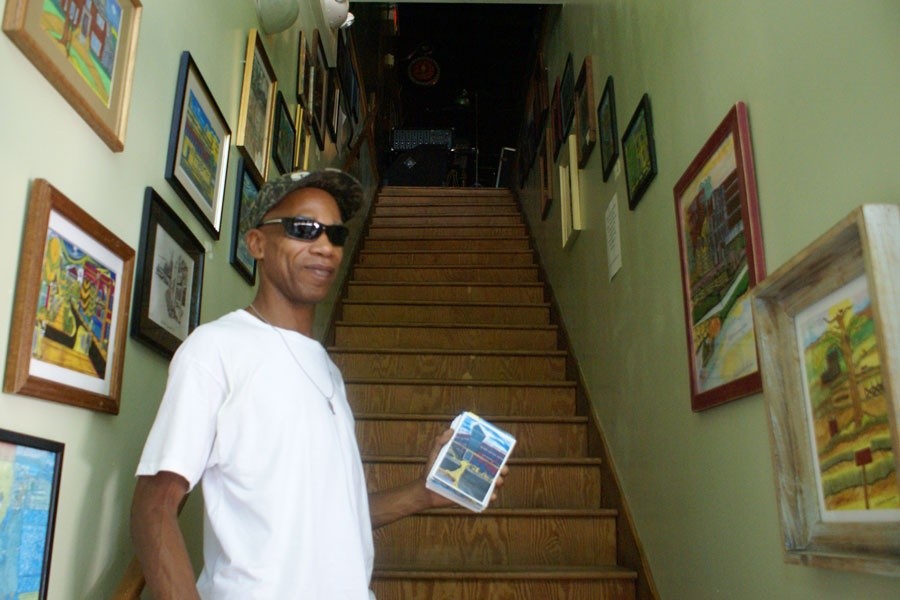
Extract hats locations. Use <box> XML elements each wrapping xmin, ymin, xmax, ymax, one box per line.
<box><xmin>250</xmin><ymin>167</ymin><xmax>365</xmax><ymax>229</ymax></box>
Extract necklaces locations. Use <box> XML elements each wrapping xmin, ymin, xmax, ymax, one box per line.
<box><xmin>248</xmin><ymin>303</ymin><xmax>337</xmax><ymax>415</ymax></box>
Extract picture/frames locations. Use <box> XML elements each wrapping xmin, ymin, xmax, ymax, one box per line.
<box><xmin>0</xmin><ymin>1</ymin><xmax>359</xmax><ymax>600</ymax></box>
<box><xmin>674</xmin><ymin>101</ymin><xmax>766</xmax><ymax>412</ymax></box>
<box><xmin>598</xmin><ymin>75</ymin><xmax>619</xmax><ymax>182</ymax></box>
<box><xmin>518</xmin><ymin>52</ymin><xmax>597</xmax><ymax>221</ymax></box>
<box><xmin>750</xmin><ymin>204</ymin><xmax>900</xmax><ymax>575</ymax></box>
<box><xmin>622</xmin><ymin>92</ymin><xmax>658</xmax><ymax>211</ymax></box>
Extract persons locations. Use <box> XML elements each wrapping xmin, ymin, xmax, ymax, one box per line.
<box><xmin>129</xmin><ymin>167</ymin><xmax>378</xmax><ymax>600</ymax></box>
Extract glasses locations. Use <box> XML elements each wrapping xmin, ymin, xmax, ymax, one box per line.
<box><xmin>258</xmin><ymin>216</ymin><xmax>349</xmax><ymax>247</ymax></box>
